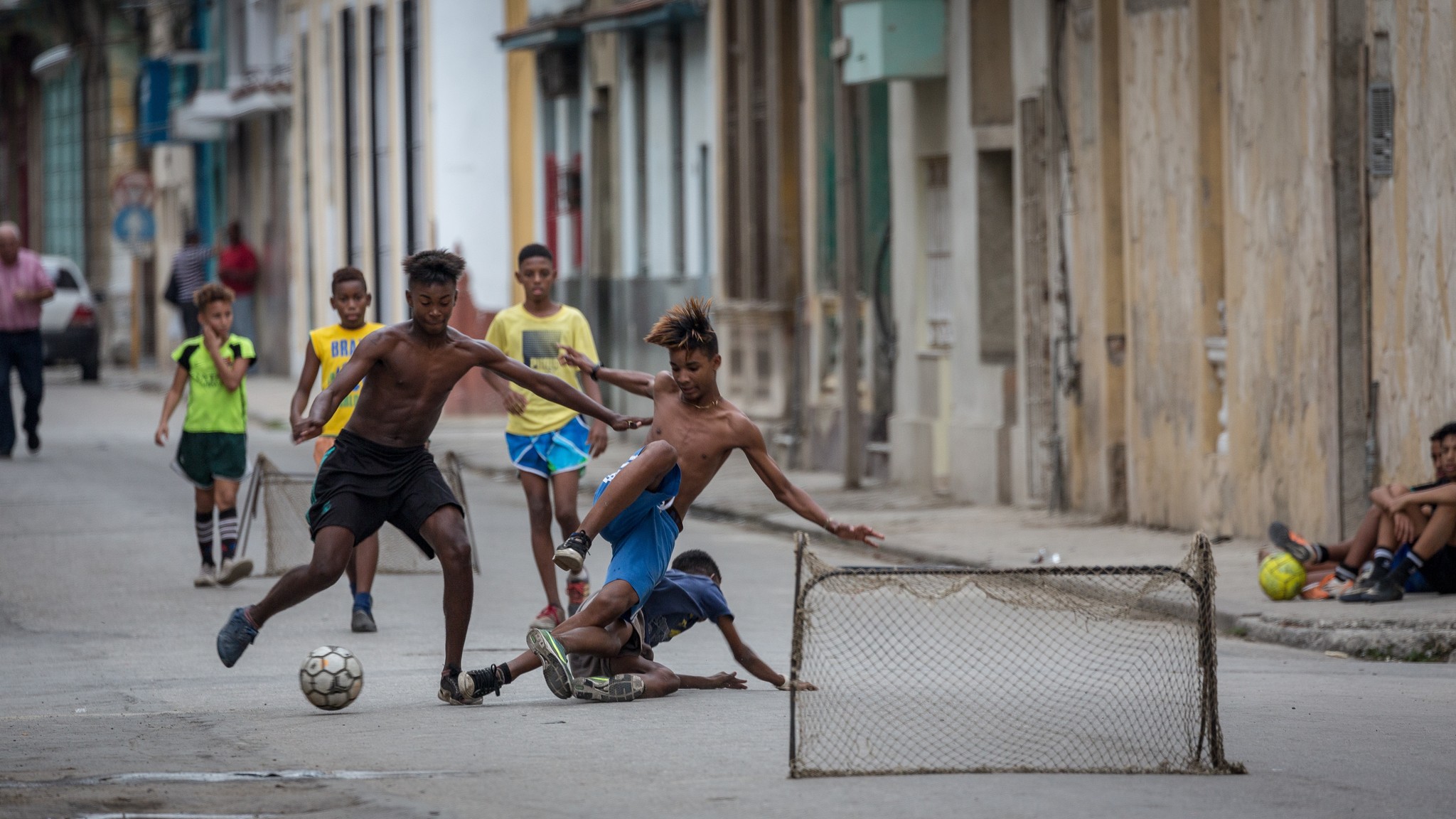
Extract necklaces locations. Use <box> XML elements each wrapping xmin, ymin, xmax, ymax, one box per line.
<box><xmin>680</xmin><ymin>394</ymin><xmax>719</xmax><ymax>409</ymax></box>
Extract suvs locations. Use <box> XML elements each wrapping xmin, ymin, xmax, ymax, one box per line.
<box><xmin>39</xmin><ymin>255</ymin><xmax>105</xmax><ymax>384</ymax></box>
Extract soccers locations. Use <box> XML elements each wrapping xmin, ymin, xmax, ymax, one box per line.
<box><xmin>1256</xmin><ymin>551</ymin><xmax>1307</xmax><ymax>601</ymax></box>
<box><xmin>298</xmin><ymin>644</ymin><xmax>363</xmax><ymax>710</ymax></box>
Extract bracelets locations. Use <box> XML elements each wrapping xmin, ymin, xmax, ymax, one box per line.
<box><xmin>824</xmin><ymin>518</ymin><xmax>832</xmax><ymax>529</ymax></box>
<box><xmin>591</xmin><ymin>364</ymin><xmax>601</xmax><ymax>381</ymax></box>
<box><xmin>774</xmin><ymin>673</ymin><xmax>789</xmax><ymax>689</ymax></box>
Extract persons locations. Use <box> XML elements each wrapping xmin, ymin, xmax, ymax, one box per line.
<box><xmin>171</xmin><ymin>226</ymin><xmax>221</xmax><ymax>338</ymax></box>
<box><xmin>1269</xmin><ymin>421</ymin><xmax>1456</xmax><ymax>603</ymax></box>
<box><xmin>526</xmin><ymin>549</ymin><xmax>819</xmax><ymax>702</ymax></box>
<box><xmin>0</xmin><ymin>223</ymin><xmax>55</xmax><ymax>458</ymax></box>
<box><xmin>217</xmin><ymin>249</ymin><xmax>655</xmax><ymax>705</ymax></box>
<box><xmin>155</xmin><ymin>282</ymin><xmax>258</xmax><ymax>589</ymax></box>
<box><xmin>215</xmin><ymin>220</ymin><xmax>260</xmax><ymax>343</ymax></box>
<box><xmin>290</xmin><ymin>265</ymin><xmax>390</xmax><ymax>632</ymax></box>
<box><xmin>457</xmin><ymin>297</ymin><xmax>884</xmax><ymax>699</ymax></box>
<box><xmin>479</xmin><ymin>244</ymin><xmax>607</xmax><ymax>630</ymax></box>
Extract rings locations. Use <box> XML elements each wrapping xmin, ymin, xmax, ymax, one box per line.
<box><xmin>628</xmin><ymin>421</ymin><xmax>630</xmax><ymax>429</ymax></box>
<box><xmin>565</xmin><ymin>355</ymin><xmax>568</xmax><ymax>359</ymax></box>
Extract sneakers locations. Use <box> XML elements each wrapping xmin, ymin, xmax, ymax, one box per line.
<box><xmin>194</xmin><ymin>564</ymin><xmax>217</xmax><ymax>585</ymax></box>
<box><xmin>553</xmin><ymin>529</ymin><xmax>592</xmax><ymax>571</ymax></box>
<box><xmin>437</xmin><ymin>668</ymin><xmax>484</xmax><ymax>706</ymax></box>
<box><xmin>1340</xmin><ymin>573</ymin><xmax>1384</xmax><ymax>601</ymax></box>
<box><xmin>1300</xmin><ymin>571</ymin><xmax>1357</xmax><ymax>601</ymax></box>
<box><xmin>1361</xmin><ymin>578</ymin><xmax>1403</xmax><ymax>601</ymax></box>
<box><xmin>458</xmin><ymin>663</ymin><xmax>505</xmax><ymax>701</ymax></box>
<box><xmin>530</xmin><ymin>604</ymin><xmax>565</xmax><ymax>631</ymax></box>
<box><xmin>217</xmin><ymin>558</ymin><xmax>253</xmax><ymax>587</ymax></box>
<box><xmin>351</xmin><ymin>608</ymin><xmax>377</xmax><ymax>632</ymax></box>
<box><xmin>216</xmin><ymin>608</ymin><xmax>258</xmax><ymax>668</ymax></box>
<box><xmin>526</xmin><ymin>628</ymin><xmax>574</xmax><ymax>700</ymax></box>
<box><xmin>1267</xmin><ymin>522</ymin><xmax>1319</xmax><ymax>566</ymax></box>
<box><xmin>573</xmin><ymin>673</ymin><xmax>644</xmax><ymax>702</ymax></box>
<box><xmin>566</xmin><ymin>566</ymin><xmax>590</xmax><ymax>617</ymax></box>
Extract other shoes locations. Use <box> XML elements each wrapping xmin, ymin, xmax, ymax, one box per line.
<box><xmin>0</xmin><ymin>449</ymin><xmax>11</xmax><ymax>459</ymax></box>
<box><xmin>24</xmin><ymin>428</ymin><xmax>40</xmax><ymax>451</ymax></box>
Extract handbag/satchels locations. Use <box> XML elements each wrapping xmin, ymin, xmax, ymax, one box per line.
<box><xmin>164</xmin><ymin>274</ymin><xmax>179</xmax><ymax>307</ymax></box>
